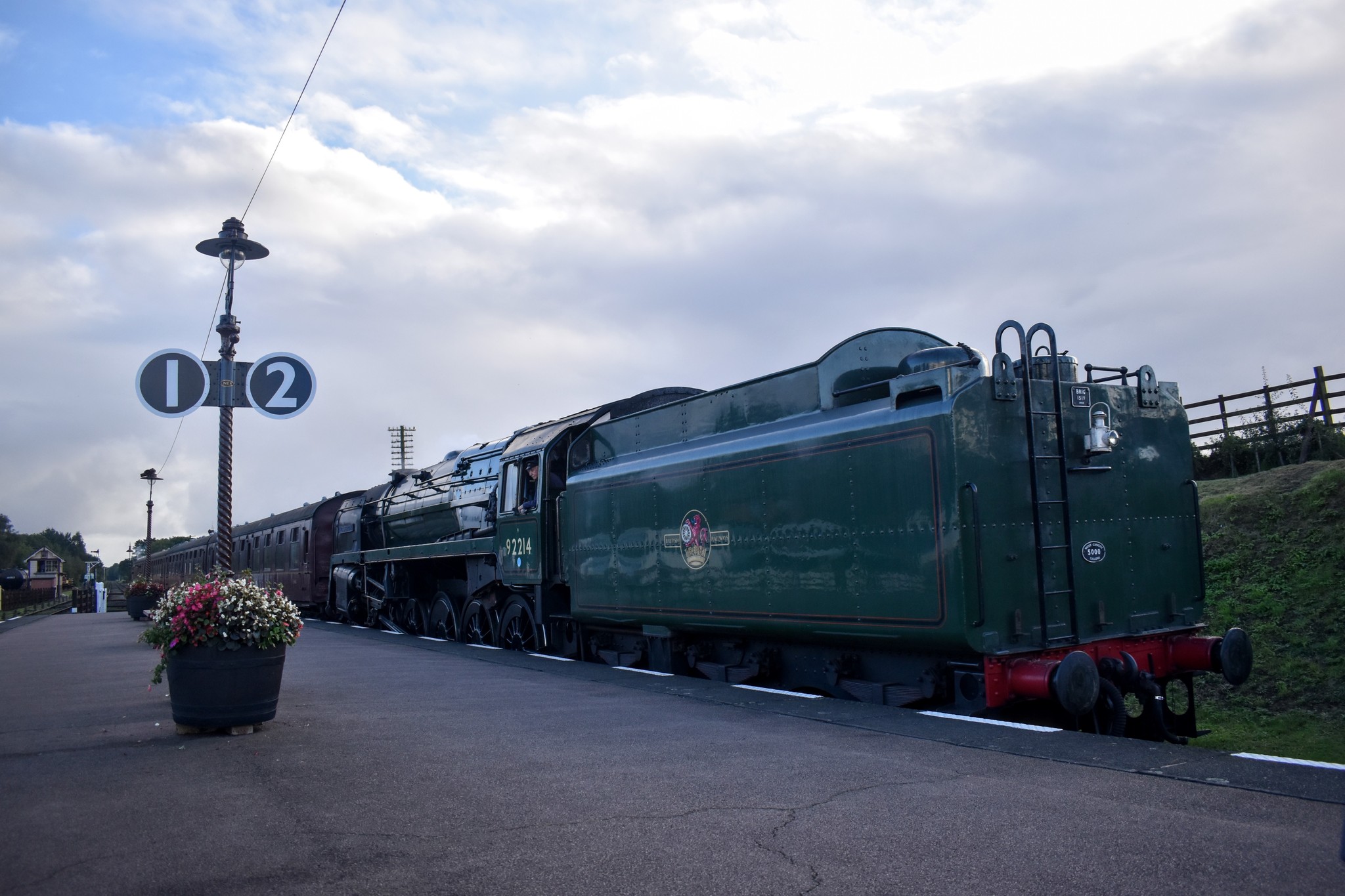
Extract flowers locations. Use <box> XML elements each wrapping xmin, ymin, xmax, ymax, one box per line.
<box><xmin>126</xmin><ymin>577</ymin><xmax>169</xmax><ymax>597</ymax></box>
<box><xmin>137</xmin><ymin>570</ymin><xmax>302</xmax><ymax>687</ymax></box>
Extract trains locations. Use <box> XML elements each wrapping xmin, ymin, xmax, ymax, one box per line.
<box><xmin>0</xmin><ymin>568</ymin><xmax>74</xmax><ymax>600</ymax></box>
<box><xmin>116</xmin><ymin>318</ymin><xmax>1255</xmax><ymax>750</ymax></box>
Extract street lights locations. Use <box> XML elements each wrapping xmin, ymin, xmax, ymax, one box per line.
<box><xmin>192</xmin><ymin>217</ymin><xmax>272</xmax><ymax>576</ymax></box>
<box><xmin>139</xmin><ymin>468</ymin><xmax>164</xmax><ymax>582</ymax></box>
<box><xmin>126</xmin><ymin>542</ymin><xmax>134</xmax><ymax>560</ymax></box>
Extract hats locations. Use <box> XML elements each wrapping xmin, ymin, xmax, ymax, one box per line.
<box><xmin>526</xmin><ymin>460</ymin><xmax>539</xmax><ymax>470</ymax></box>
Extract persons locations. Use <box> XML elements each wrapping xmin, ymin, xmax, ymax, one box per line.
<box><xmin>518</xmin><ymin>460</ymin><xmax>564</xmax><ymax>514</ymax></box>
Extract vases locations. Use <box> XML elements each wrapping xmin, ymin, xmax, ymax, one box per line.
<box><xmin>166</xmin><ymin>637</ymin><xmax>290</xmax><ymax>727</ymax></box>
<box><xmin>128</xmin><ymin>591</ymin><xmax>161</xmax><ymax>621</ymax></box>
